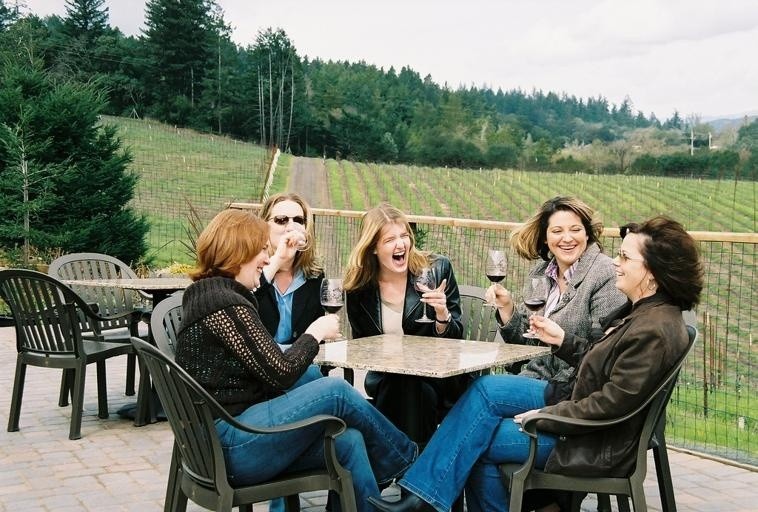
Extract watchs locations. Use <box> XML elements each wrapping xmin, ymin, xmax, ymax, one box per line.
<box><xmin>433</xmin><ymin>313</ymin><xmax>453</xmax><ymax>326</ymax></box>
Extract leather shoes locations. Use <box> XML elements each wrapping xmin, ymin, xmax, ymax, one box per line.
<box><xmin>367</xmin><ymin>493</ymin><xmax>437</xmax><ymax>512</ymax></box>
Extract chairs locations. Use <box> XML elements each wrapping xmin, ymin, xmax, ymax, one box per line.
<box><xmin>129</xmin><ymin>335</ymin><xmax>357</xmax><ymax>511</ymax></box>
<box><xmin>498</xmin><ymin>324</ymin><xmax>698</xmax><ymax>512</ymax></box>
<box><xmin>0</xmin><ymin>267</ymin><xmax>144</xmax><ymax>441</ymax></box>
<box><xmin>151</xmin><ymin>295</ymin><xmax>337</xmax><ymax>512</ymax></box>
<box><xmin>504</xmin><ymin>359</ymin><xmax>677</xmax><ymax>512</ymax></box>
<box><xmin>342</xmin><ymin>285</ymin><xmax>506</xmax><ymax>387</ymax></box>
<box><xmin>47</xmin><ymin>252</ymin><xmax>153</xmax><ymax>407</ymax></box>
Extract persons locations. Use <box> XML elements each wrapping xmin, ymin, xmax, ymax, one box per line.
<box><xmin>482</xmin><ymin>195</ymin><xmax>628</xmax><ymax>379</ymax></box>
<box><xmin>257</xmin><ymin>194</ymin><xmax>328</xmax><ymax>346</ymax></box>
<box><xmin>366</xmin><ymin>217</ymin><xmax>704</xmax><ymax>512</ymax></box>
<box><xmin>175</xmin><ymin>209</ymin><xmax>421</xmax><ymax>512</ymax></box>
<box><xmin>343</xmin><ymin>205</ymin><xmax>463</xmax><ymax>453</ymax></box>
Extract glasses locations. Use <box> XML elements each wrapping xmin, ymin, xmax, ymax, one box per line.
<box><xmin>619</xmin><ymin>249</ymin><xmax>632</xmax><ymax>263</ymax></box>
<box><xmin>268</xmin><ymin>215</ymin><xmax>305</xmax><ymax>225</ymax></box>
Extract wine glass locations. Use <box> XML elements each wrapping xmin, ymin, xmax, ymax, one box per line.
<box><xmin>414</xmin><ymin>268</ymin><xmax>437</xmax><ymax>323</ymax></box>
<box><xmin>523</xmin><ymin>276</ymin><xmax>549</xmax><ymax>337</ymax></box>
<box><xmin>482</xmin><ymin>251</ymin><xmax>509</xmax><ymax>307</ymax></box>
<box><xmin>286</xmin><ymin>223</ymin><xmax>313</xmax><ymax>251</ymax></box>
<box><xmin>321</xmin><ymin>279</ymin><xmax>346</xmax><ymax>341</ymax></box>
